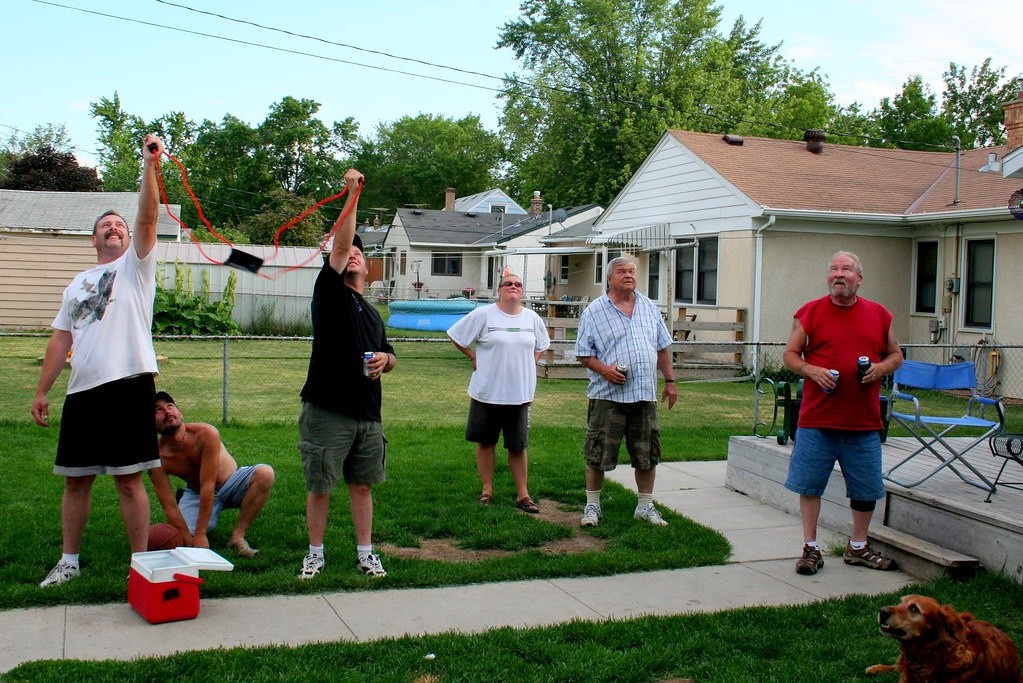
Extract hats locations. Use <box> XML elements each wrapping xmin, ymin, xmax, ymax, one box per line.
<box><xmin>352</xmin><ymin>234</ymin><xmax>364</xmax><ymax>253</ymax></box>
<box><xmin>156</xmin><ymin>390</ymin><xmax>175</xmax><ymax>404</ymax></box>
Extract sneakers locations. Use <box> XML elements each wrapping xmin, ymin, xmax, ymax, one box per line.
<box><xmin>634</xmin><ymin>502</ymin><xmax>669</xmax><ymax>527</ymax></box>
<box><xmin>40</xmin><ymin>559</ymin><xmax>80</xmax><ymax>588</ymax></box>
<box><xmin>357</xmin><ymin>552</ymin><xmax>387</xmax><ymax>578</ymax></box>
<box><xmin>298</xmin><ymin>553</ymin><xmax>325</xmax><ymax>580</ymax></box>
<box><xmin>227</xmin><ymin>537</ymin><xmax>258</xmax><ymax>557</ymax></box>
<box><xmin>580</xmin><ymin>503</ymin><xmax>603</xmax><ymax>527</ymax></box>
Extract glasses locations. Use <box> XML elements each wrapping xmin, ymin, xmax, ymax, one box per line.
<box><xmin>500</xmin><ymin>280</ymin><xmax>523</xmax><ymax>289</ymax></box>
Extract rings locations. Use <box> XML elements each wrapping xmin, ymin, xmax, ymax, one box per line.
<box><xmin>874</xmin><ymin>375</ymin><xmax>876</xmax><ymax>377</ymax></box>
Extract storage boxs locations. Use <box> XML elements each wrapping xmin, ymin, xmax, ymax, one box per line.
<box><xmin>127</xmin><ymin>543</ymin><xmax>234</xmax><ymax>622</ymax></box>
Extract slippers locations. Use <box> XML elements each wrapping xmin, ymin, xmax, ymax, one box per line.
<box><xmin>517</xmin><ymin>495</ymin><xmax>539</xmax><ymax>513</ymax></box>
<box><xmin>480</xmin><ymin>494</ymin><xmax>492</xmax><ymax>506</ymax></box>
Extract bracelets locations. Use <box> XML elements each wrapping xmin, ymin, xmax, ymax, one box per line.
<box><xmin>665</xmin><ymin>380</ymin><xmax>674</xmax><ymax>383</ymax></box>
<box><xmin>470</xmin><ymin>357</ymin><xmax>473</xmax><ymax>360</ymax></box>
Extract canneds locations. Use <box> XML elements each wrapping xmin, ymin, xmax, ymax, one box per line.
<box><xmin>616</xmin><ymin>364</ymin><xmax>628</xmax><ymax>380</ymax></box>
<box><xmin>362</xmin><ymin>352</ymin><xmax>376</xmax><ymax>378</ymax></box>
<box><xmin>822</xmin><ymin>369</ymin><xmax>839</xmax><ymax>393</ymax></box>
<box><xmin>857</xmin><ymin>356</ymin><xmax>871</xmax><ymax>382</ymax></box>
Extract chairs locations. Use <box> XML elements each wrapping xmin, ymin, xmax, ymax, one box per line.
<box><xmin>530</xmin><ymin>295</ymin><xmax>581</xmax><ymax>318</ymax></box>
<box><xmin>672</xmin><ymin>314</ymin><xmax>697</xmax><ymax>363</ymax></box>
<box><xmin>883</xmin><ymin>361</ymin><xmax>1006</xmax><ymax>489</ymax></box>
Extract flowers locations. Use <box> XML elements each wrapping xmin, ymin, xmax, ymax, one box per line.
<box><xmin>463</xmin><ymin>287</ymin><xmax>475</xmax><ymax>298</ymax></box>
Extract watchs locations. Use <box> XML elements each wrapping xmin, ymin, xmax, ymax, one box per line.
<box><xmin>386</xmin><ymin>353</ymin><xmax>391</xmax><ymax>365</ymax></box>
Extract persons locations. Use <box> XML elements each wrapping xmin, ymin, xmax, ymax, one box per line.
<box><xmin>32</xmin><ymin>135</ymin><xmax>166</xmax><ymax>590</ymax></box>
<box><xmin>447</xmin><ymin>275</ymin><xmax>551</xmax><ymax>512</ymax></box>
<box><xmin>295</xmin><ymin>170</ymin><xmax>396</xmax><ymax>580</ymax></box>
<box><xmin>575</xmin><ymin>257</ymin><xmax>677</xmax><ymax>528</ymax></box>
<box><xmin>148</xmin><ymin>392</ymin><xmax>276</xmax><ymax>557</ymax></box>
<box><xmin>781</xmin><ymin>252</ymin><xmax>903</xmax><ymax>576</ymax></box>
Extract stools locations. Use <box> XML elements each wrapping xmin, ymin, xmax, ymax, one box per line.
<box><xmin>984</xmin><ymin>433</ymin><xmax>1023</xmax><ymax>503</ymax></box>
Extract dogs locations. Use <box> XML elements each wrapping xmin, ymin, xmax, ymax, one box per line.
<box><xmin>867</xmin><ymin>594</ymin><xmax>1023</xmax><ymax>683</ymax></box>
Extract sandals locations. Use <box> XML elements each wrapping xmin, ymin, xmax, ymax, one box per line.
<box><xmin>843</xmin><ymin>536</ymin><xmax>898</xmax><ymax>571</ymax></box>
<box><xmin>795</xmin><ymin>542</ymin><xmax>824</xmax><ymax>575</ymax></box>
